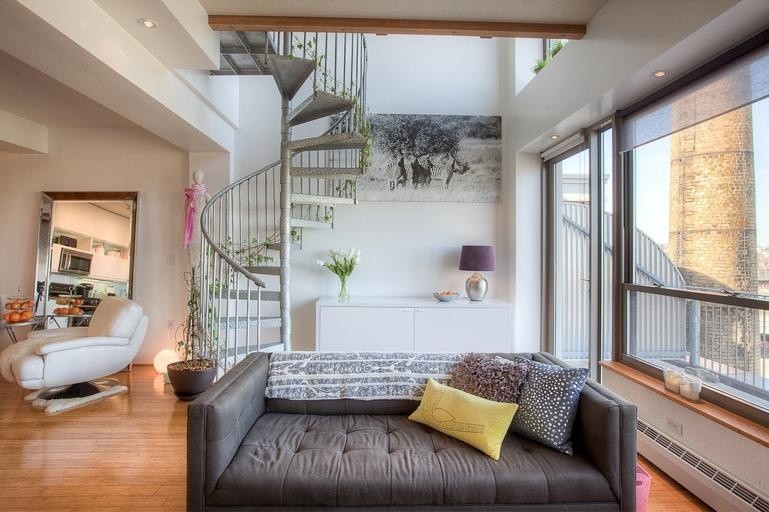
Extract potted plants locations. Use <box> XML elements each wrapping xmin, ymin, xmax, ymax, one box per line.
<box><xmin>167</xmin><ymin>266</ymin><xmax>218</xmax><ymax>402</ymax></box>
<box><xmin>532</xmin><ymin>57</ymin><xmax>547</xmax><ymax>73</ymax></box>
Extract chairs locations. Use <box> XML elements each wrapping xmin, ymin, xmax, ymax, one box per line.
<box><xmin>0</xmin><ymin>295</ymin><xmax>149</xmax><ymax>415</ymax></box>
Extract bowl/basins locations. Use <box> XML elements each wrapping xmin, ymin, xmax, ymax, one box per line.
<box><xmin>432</xmin><ymin>292</ymin><xmax>459</xmax><ymax>302</ymax></box>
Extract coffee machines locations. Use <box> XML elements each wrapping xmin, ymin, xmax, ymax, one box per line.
<box><xmin>77</xmin><ymin>282</ymin><xmax>100</xmax><ymax>305</ymax></box>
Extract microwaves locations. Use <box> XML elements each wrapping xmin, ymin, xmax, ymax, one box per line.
<box><xmin>59</xmin><ymin>248</ymin><xmax>91</xmax><ymax>275</ymax></box>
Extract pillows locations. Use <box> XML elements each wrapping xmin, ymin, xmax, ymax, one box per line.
<box><xmin>406</xmin><ymin>353</ymin><xmax>591</xmax><ymax>461</ymax></box>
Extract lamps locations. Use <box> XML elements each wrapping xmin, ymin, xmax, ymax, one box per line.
<box><xmin>152</xmin><ymin>349</ymin><xmax>180</xmax><ymax>385</ymax></box>
<box><xmin>459</xmin><ymin>246</ymin><xmax>496</xmax><ymax>302</ymax></box>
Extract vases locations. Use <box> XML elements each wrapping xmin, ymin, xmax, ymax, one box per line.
<box><xmin>337</xmin><ymin>274</ymin><xmax>350</xmax><ymax>302</ymax></box>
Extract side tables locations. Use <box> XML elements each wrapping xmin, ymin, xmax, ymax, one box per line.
<box><xmin>0</xmin><ymin>316</ymin><xmax>44</xmax><ymax>344</ymax></box>
<box><xmin>47</xmin><ymin>314</ymin><xmax>92</xmax><ymax>328</ymax></box>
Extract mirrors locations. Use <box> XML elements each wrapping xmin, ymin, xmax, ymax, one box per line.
<box><xmin>31</xmin><ymin>192</ymin><xmax>136</xmax><ymax>327</ymax></box>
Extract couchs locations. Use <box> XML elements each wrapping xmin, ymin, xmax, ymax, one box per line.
<box><xmin>186</xmin><ymin>350</ymin><xmax>636</xmax><ymax>512</ymax></box>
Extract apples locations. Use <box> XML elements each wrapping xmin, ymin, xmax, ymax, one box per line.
<box><xmin>56</xmin><ymin>297</ymin><xmax>82</xmax><ymax>305</ymax></box>
<box><xmin>6</xmin><ymin>300</ymin><xmax>33</xmax><ymax>310</ymax></box>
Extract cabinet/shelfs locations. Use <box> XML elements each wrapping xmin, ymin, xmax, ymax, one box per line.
<box><xmin>47</xmin><ymin>225</ymin><xmax>130</xmax><ymax>282</ymax></box>
<box><xmin>315</xmin><ymin>297</ymin><xmax>512</xmax><ymax>353</ymax></box>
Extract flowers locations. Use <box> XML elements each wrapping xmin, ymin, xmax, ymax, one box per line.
<box><xmin>316</xmin><ymin>245</ymin><xmax>361</xmax><ymax>295</ymax></box>
